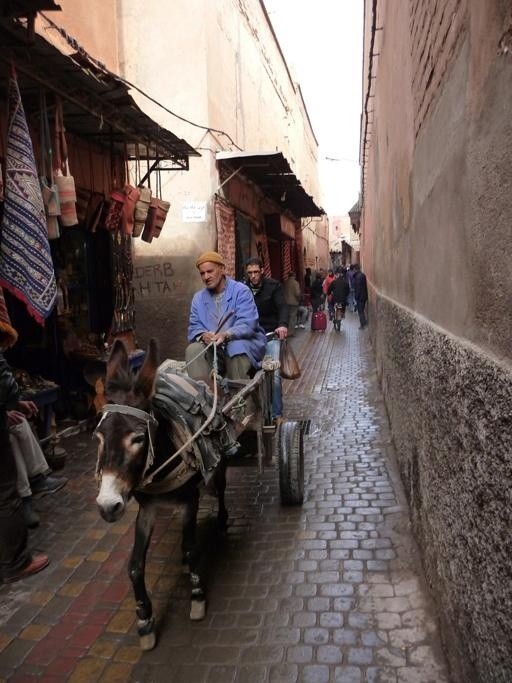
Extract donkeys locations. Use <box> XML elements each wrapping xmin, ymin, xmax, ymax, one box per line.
<box><xmin>92</xmin><ymin>338</ymin><xmax>228</xmax><ymax>652</ymax></box>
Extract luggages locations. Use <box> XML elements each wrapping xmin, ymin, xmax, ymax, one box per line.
<box><xmin>310</xmin><ymin>311</ymin><xmax>327</xmax><ymax>332</ymax></box>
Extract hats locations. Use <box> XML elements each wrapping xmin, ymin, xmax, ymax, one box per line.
<box><xmin>196</xmin><ymin>251</ymin><xmax>226</xmax><ymax>268</ymax></box>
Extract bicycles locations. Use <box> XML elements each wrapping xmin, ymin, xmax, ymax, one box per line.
<box><xmin>332</xmin><ymin>302</ymin><xmax>344</xmax><ymax>332</ymax></box>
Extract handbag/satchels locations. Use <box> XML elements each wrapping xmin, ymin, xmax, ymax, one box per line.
<box><xmin>280</xmin><ymin>337</ymin><xmax>301</xmax><ymax>380</ymax></box>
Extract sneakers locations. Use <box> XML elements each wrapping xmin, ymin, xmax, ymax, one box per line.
<box><xmin>330</xmin><ymin>311</ymin><xmax>344</xmax><ymax>319</ymax></box>
<box><xmin>30</xmin><ymin>477</ymin><xmax>69</xmax><ymax>499</ymax></box>
<box><xmin>359</xmin><ymin>320</ymin><xmax>368</xmax><ymax>329</ymax></box>
<box><xmin>288</xmin><ymin>323</ymin><xmax>304</xmax><ymax>338</ymax></box>
<box><xmin>4</xmin><ymin>553</ymin><xmax>51</xmax><ymax>586</ymax></box>
<box><xmin>229</xmin><ymin>396</ymin><xmax>248</xmax><ymax>420</ymax></box>
<box><xmin>19</xmin><ymin>499</ymin><xmax>40</xmax><ymax>526</ymax></box>
<box><xmin>273</xmin><ymin>416</ymin><xmax>286</xmax><ymax>426</ymax></box>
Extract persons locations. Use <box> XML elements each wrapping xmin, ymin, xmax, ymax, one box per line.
<box><xmin>282</xmin><ymin>271</ymin><xmax>302</xmax><ymax>336</ymax></box>
<box><xmin>184</xmin><ymin>249</ymin><xmax>268</xmax><ymax>404</ymax></box>
<box><xmin>1</xmin><ymin>399</ymin><xmax>68</xmax><ymax>528</ymax></box>
<box><xmin>0</xmin><ymin>352</ymin><xmax>52</xmax><ymax>588</ymax></box>
<box><xmin>351</xmin><ymin>264</ymin><xmax>368</xmax><ymax>330</ymax></box>
<box><xmin>241</xmin><ymin>256</ymin><xmax>290</xmax><ymax>425</ymax></box>
<box><xmin>304</xmin><ymin>262</ymin><xmax>356</xmax><ymax>321</ymax></box>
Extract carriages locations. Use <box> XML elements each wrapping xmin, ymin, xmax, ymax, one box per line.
<box><xmin>88</xmin><ymin>336</ymin><xmax>304</xmax><ymax>652</ymax></box>
